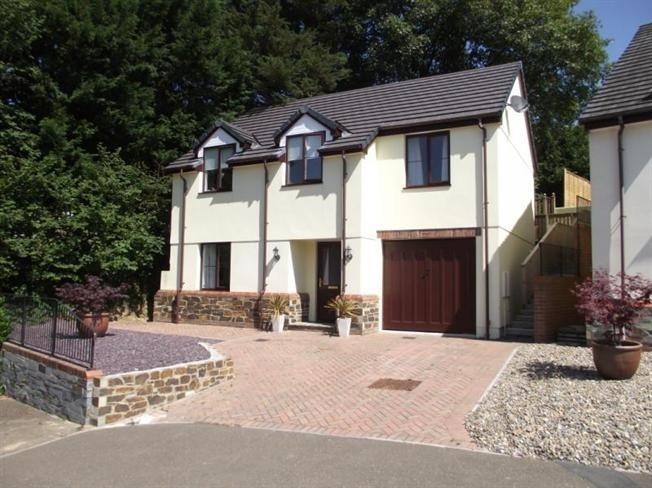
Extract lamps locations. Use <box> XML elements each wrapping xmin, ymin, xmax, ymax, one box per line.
<box><xmin>273</xmin><ymin>246</ymin><xmax>280</xmax><ymax>261</ymax></box>
<box><xmin>345</xmin><ymin>245</ymin><xmax>352</xmax><ymax>261</ymax></box>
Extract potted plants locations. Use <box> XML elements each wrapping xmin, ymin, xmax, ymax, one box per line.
<box><xmin>569</xmin><ymin>269</ymin><xmax>652</xmax><ymax>380</ymax></box>
<box><xmin>53</xmin><ymin>273</ymin><xmax>131</xmax><ymax>337</ymax></box>
<box><xmin>263</xmin><ymin>294</ymin><xmax>291</xmax><ymax>333</ymax></box>
<box><xmin>323</xmin><ymin>294</ymin><xmax>360</xmax><ymax>338</ymax></box>
